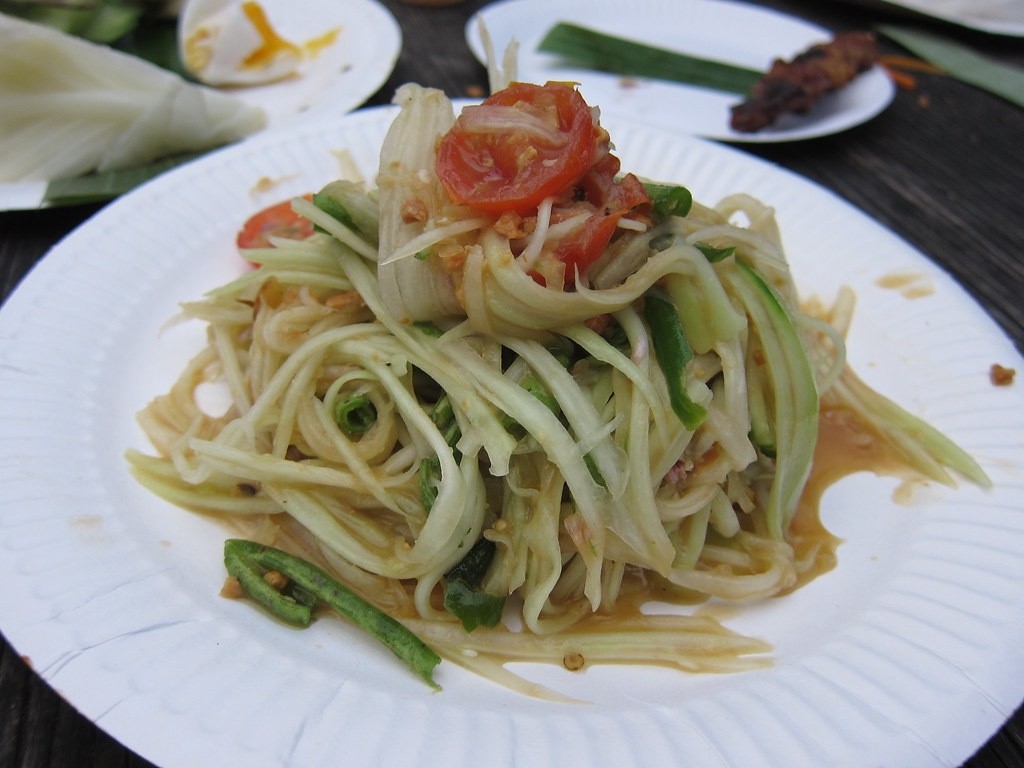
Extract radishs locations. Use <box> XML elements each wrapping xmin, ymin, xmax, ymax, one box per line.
<box><xmin>120</xmin><ymin>177</ymin><xmax>990</xmax><ymax>702</ymax></box>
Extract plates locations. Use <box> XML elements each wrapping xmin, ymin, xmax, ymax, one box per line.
<box><xmin>465</xmin><ymin>0</ymin><xmax>896</xmax><ymax>142</ymax></box>
<box><xmin>1</xmin><ymin>101</ymin><xmax>1023</xmax><ymax>767</ymax></box>
<box><xmin>1</xmin><ymin>0</ymin><xmax>403</xmax><ymax>213</ymax></box>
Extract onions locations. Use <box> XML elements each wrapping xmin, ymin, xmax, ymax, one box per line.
<box><xmin>378</xmin><ymin>83</ymin><xmax>495</xmax><ymax>324</ymax></box>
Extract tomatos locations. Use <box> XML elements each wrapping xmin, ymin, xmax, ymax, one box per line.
<box><xmin>532</xmin><ymin>203</ymin><xmax>627</xmax><ymax>285</ymax></box>
<box><xmin>236</xmin><ymin>193</ymin><xmax>315</xmax><ymax>264</ymax></box>
<box><xmin>432</xmin><ymin>80</ymin><xmax>599</xmax><ymax>212</ymax></box>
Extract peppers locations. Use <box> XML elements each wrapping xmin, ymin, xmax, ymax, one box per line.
<box><xmin>644</xmin><ymin>289</ymin><xmax>708</xmax><ymax>430</ymax></box>
<box><xmin>416</xmin><ymin>458</ymin><xmax>508</xmax><ymax>631</ymax></box>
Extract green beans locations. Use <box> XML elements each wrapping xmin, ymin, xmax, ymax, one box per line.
<box><xmin>223</xmin><ymin>535</ymin><xmax>443</xmax><ymax>688</ymax></box>
<box><xmin>428</xmin><ymin>392</ymin><xmax>454</xmax><ymax>430</ymax></box>
<box><xmin>332</xmin><ymin>394</ymin><xmax>375</xmax><ymax>435</ymax></box>
<box><xmin>224</xmin><ymin>550</ymin><xmax>311</xmax><ymax>626</ymax></box>
<box><xmin>638</xmin><ymin>181</ymin><xmax>693</xmax><ymax>220</ymax></box>
